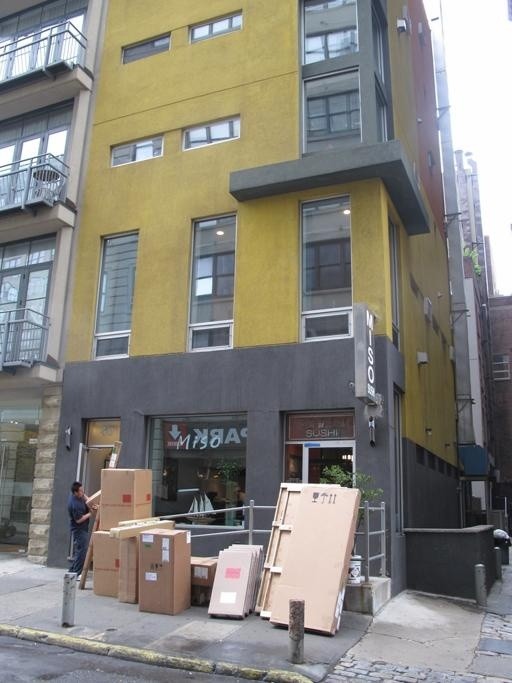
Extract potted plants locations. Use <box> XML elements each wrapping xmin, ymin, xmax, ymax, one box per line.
<box><xmin>319</xmin><ymin>464</ymin><xmax>385</xmax><ymax>583</ymax></box>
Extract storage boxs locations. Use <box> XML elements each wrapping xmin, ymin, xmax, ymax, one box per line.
<box><xmin>92</xmin><ymin>468</ymin><xmax>219</xmax><ymax>616</ymax></box>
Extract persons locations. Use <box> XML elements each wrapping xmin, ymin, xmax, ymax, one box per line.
<box><xmin>67</xmin><ymin>482</ymin><xmax>99</xmax><ymax>581</ymax></box>
